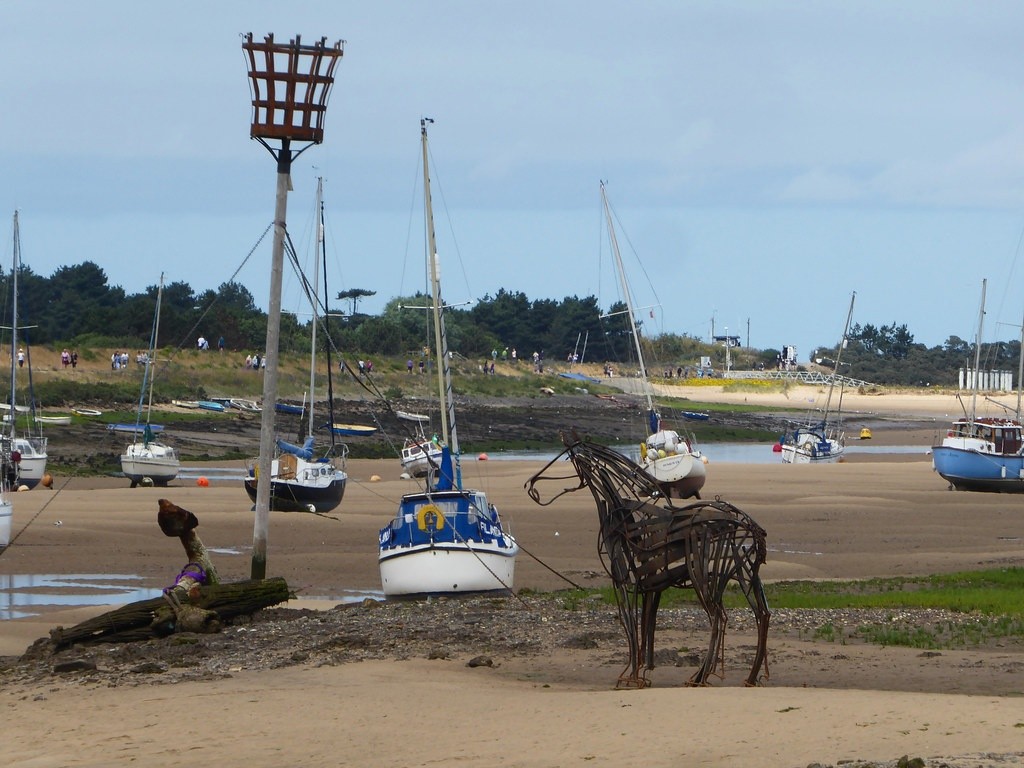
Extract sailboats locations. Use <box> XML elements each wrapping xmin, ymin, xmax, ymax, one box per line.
<box><xmin>242</xmin><ymin>178</ymin><xmax>350</xmax><ymax>513</ymax></box>
<box><xmin>120</xmin><ymin>271</ymin><xmax>180</xmax><ymax>487</ymax></box>
<box><xmin>0</xmin><ymin>209</ymin><xmax>50</xmax><ymax>490</ymax></box>
<box><xmin>379</xmin><ymin>116</ymin><xmax>518</xmax><ymax>601</ymax></box>
<box><xmin>596</xmin><ymin>179</ymin><xmax>710</xmax><ymax>499</ymax></box>
<box><xmin>773</xmin><ymin>289</ymin><xmax>858</xmax><ymax>465</ymax></box>
<box><xmin>926</xmin><ymin>229</ymin><xmax>1024</xmax><ymax>494</ymax></box>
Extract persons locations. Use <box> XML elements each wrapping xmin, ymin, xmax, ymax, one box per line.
<box><xmin>604</xmin><ymin>363</ymin><xmax>613</xmax><ymax>378</ymax></box>
<box><xmin>261</xmin><ymin>353</ymin><xmax>266</xmax><ymax>367</ymax></box>
<box><xmin>218</xmin><ymin>336</ymin><xmax>225</xmax><ymax>352</ymax></box>
<box><xmin>70</xmin><ymin>350</ymin><xmax>78</xmax><ymax>368</ymax></box>
<box><xmin>751</xmin><ymin>359</ymin><xmax>785</xmax><ymax>371</ymax></box>
<box><xmin>136</xmin><ymin>351</ymin><xmax>142</xmax><ymax>364</ymax></box>
<box><xmin>252</xmin><ymin>355</ymin><xmax>257</xmax><ymax>367</ymax></box>
<box><xmin>61</xmin><ymin>349</ymin><xmax>70</xmax><ymax>368</ymax></box>
<box><xmin>197</xmin><ymin>335</ymin><xmax>209</xmax><ymax>351</ymax></box>
<box><xmin>141</xmin><ymin>350</ymin><xmax>147</xmax><ymax>366</ymax></box>
<box><xmin>111</xmin><ymin>351</ymin><xmax>129</xmax><ymax>370</ymax></box>
<box><xmin>246</xmin><ymin>355</ymin><xmax>251</xmax><ymax>369</ymax></box>
<box><xmin>491</xmin><ymin>347</ymin><xmax>517</xmax><ymax>362</ymax></box>
<box><xmin>484</xmin><ymin>360</ymin><xmax>494</xmax><ymax>375</ymax></box>
<box><xmin>533</xmin><ymin>349</ymin><xmax>544</xmax><ymax>374</ymax></box>
<box><xmin>254</xmin><ymin>354</ymin><xmax>260</xmax><ymax>369</ymax></box>
<box><xmin>697</xmin><ymin>368</ymin><xmax>714</xmax><ymax>379</ymax></box>
<box><xmin>17</xmin><ymin>349</ymin><xmax>25</xmax><ymax>366</ymax></box>
<box><xmin>567</xmin><ymin>352</ymin><xmax>578</xmax><ymax>365</ymax></box>
<box><xmin>664</xmin><ymin>366</ymin><xmax>688</xmax><ymax>379</ymax></box>
<box><xmin>339</xmin><ymin>357</ymin><xmax>371</xmax><ymax>375</ymax></box>
<box><xmin>407</xmin><ymin>346</ymin><xmax>428</xmax><ymax>375</ymax></box>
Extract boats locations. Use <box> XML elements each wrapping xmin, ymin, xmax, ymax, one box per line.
<box><xmin>396</xmin><ymin>410</ymin><xmax>430</xmax><ymax>422</ymax></box>
<box><xmin>317</xmin><ymin>423</ymin><xmax>378</xmax><ymax>436</ymax></box>
<box><xmin>0</xmin><ymin>403</ymin><xmax>30</xmax><ymax>412</ymax></box>
<box><xmin>106</xmin><ymin>423</ymin><xmax>164</xmax><ymax>434</ymax></box>
<box><xmin>199</xmin><ymin>401</ymin><xmax>224</xmax><ymax>412</ymax></box>
<box><xmin>401</xmin><ymin>411</ymin><xmax>443</xmax><ymax>478</ymax></box>
<box><xmin>230</xmin><ymin>399</ymin><xmax>263</xmax><ymax>413</ymax></box>
<box><xmin>0</xmin><ymin>397</ymin><xmax>15</xmax><ymax>547</ymax></box>
<box><xmin>275</xmin><ymin>403</ymin><xmax>306</xmax><ymax>415</ymax></box>
<box><xmin>172</xmin><ymin>399</ymin><xmax>200</xmax><ymax>409</ymax></box>
<box><xmin>71</xmin><ymin>408</ymin><xmax>102</xmax><ymax>417</ymax></box>
<box><xmin>32</xmin><ymin>416</ymin><xmax>72</xmax><ymax>426</ymax></box>
<box><xmin>211</xmin><ymin>398</ymin><xmax>231</xmax><ymax>405</ymax></box>
<box><xmin>681</xmin><ymin>411</ymin><xmax>709</xmax><ymax>420</ymax></box>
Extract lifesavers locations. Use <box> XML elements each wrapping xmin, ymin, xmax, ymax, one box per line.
<box><xmin>409</xmin><ymin>441</ymin><xmax>422</xmax><ymax>447</ymax></box>
<box><xmin>804</xmin><ymin>441</ymin><xmax>812</xmax><ymax>451</ymax></box>
<box><xmin>640</xmin><ymin>443</ymin><xmax>647</xmax><ymax>458</ymax></box>
<box><xmin>254</xmin><ymin>465</ymin><xmax>258</xmax><ymax>481</ymax></box>
<box><xmin>416</xmin><ymin>504</ymin><xmax>444</xmax><ymax>532</ymax></box>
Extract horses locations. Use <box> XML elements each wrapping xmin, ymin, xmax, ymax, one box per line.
<box><xmin>525</xmin><ymin>433</ymin><xmax>772</xmax><ymax>688</ymax></box>
